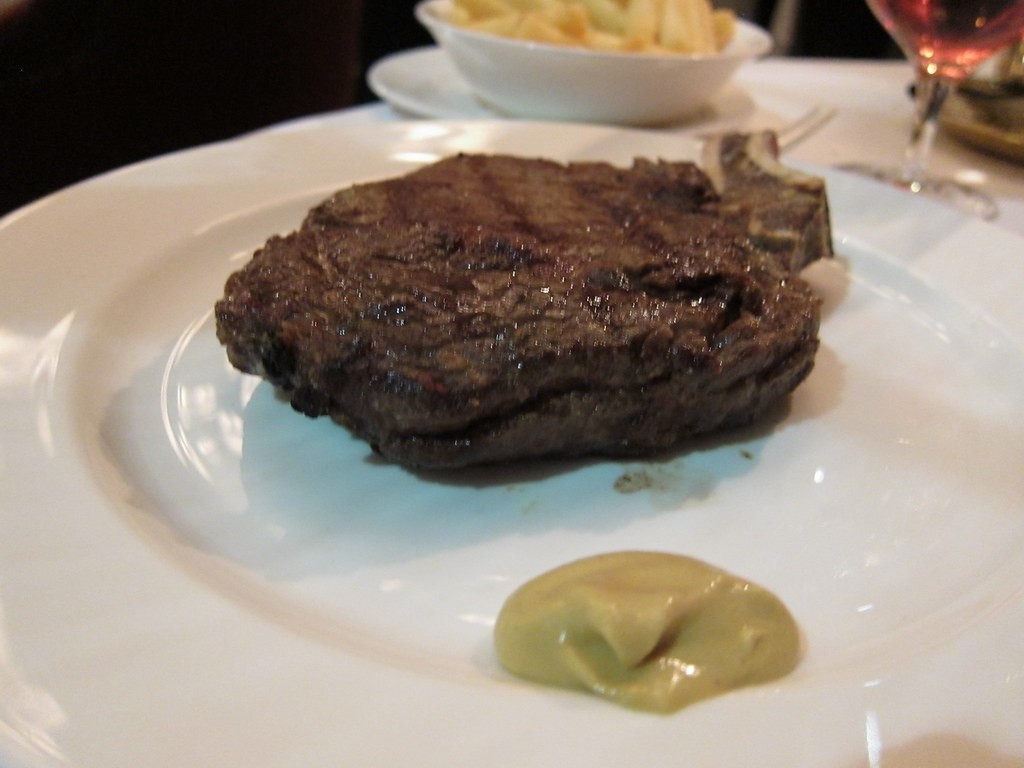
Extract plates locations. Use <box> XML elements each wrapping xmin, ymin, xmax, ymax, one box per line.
<box><xmin>2</xmin><ymin>123</ymin><xmax>1023</xmax><ymax>767</ymax></box>
<box><xmin>368</xmin><ymin>43</ymin><xmax>847</xmax><ymax>130</ymax></box>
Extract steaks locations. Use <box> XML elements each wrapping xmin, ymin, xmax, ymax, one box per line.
<box><xmin>213</xmin><ymin>130</ymin><xmax>833</xmax><ymax>474</ymax></box>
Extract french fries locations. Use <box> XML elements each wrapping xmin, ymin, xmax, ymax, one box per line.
<box><xmin>448</xmin><ymin>1</ymin><xmax>737</xmax><ymax>55</ymax></box>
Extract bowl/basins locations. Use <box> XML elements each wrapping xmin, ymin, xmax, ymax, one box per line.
<box><xmin>415</xmin><ymin>0</ymin><xmax>775</xmax><ymax>128</ymax></box>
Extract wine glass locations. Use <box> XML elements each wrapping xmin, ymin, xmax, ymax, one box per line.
<box><xmin>837</xmin><ymin>0</ymin><xmax>1024</xmax><ymax>218</ymax></box>
